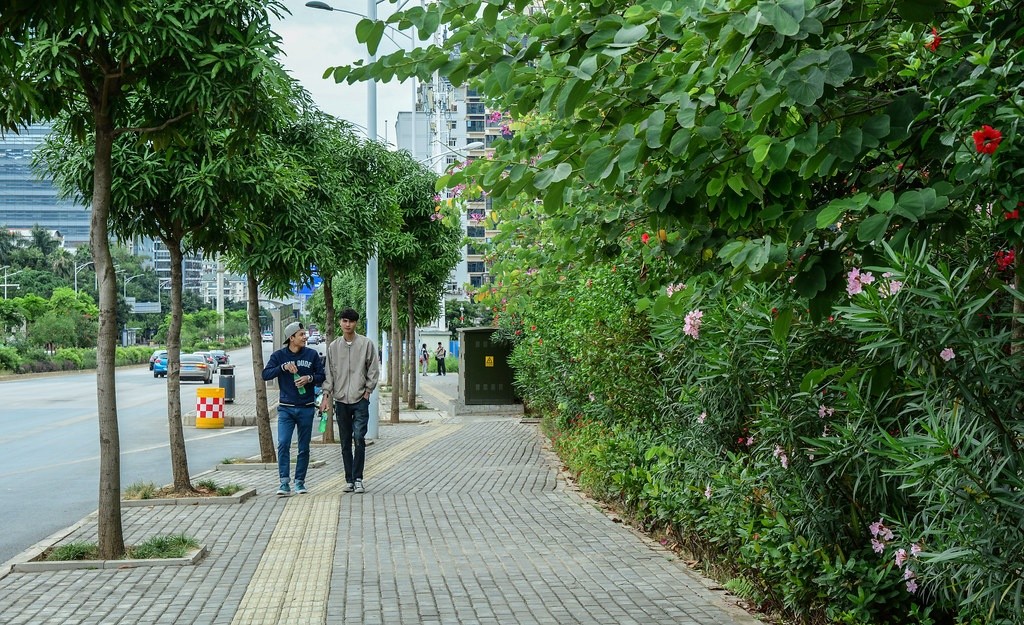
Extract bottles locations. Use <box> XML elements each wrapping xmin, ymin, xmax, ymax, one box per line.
<box><xmin>293</xmin><ymin>372</ymin><xmax>306</xmax><ymax>395</ymax></box>
<box><xmin>319</xmin><ymin>412</ymin><xmax>328</xmax><ymax>432</ymax></box>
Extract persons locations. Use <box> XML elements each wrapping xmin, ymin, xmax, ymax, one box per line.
<box><xmin>319</xmin><ymin>308</ymin><xmax>380</xmax><ymax>493</ymax></box>
<box><xmin>262</xmin><ymin>321</ymin><xmax>326</xmax><ymax>496</ymax></box>
<box><xmin>319</xmin><ymin>352</ymin><xmax>325</xmax><ymax>368</ymax></box>
<box><xmin>435</xmin><ymin>342</ymin><xmax>446</xmax><ymax>375</ymax></box>
<box><xmin>420</xmin><ymin>343</ymin><xmax>430</xmax><ymax>375</ymax></box>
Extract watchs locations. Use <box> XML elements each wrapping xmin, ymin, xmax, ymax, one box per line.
<box><xmin>307</xmin><ymin>374</ymin><xmax>313</xmax><ymax>383</ymax></box>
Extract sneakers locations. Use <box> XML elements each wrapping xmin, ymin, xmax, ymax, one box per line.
<box><xmin>354</xmin><ymin>481</ymin><xmax>365</xmax><ymax>492</ymax></box>
<box><xmin>294</xmin><ymin>479</ymin><xmax>307</xmax><ymax>494</ymax></box>
<box><xmin>343</xmin><ymin>482</ymin><xmax>355</xmax><ymax>491</ymax></box>
<box><xmin>276</xmin><ymin>483</ymin><xmax>291</xmax><ymax>495</ymax></box>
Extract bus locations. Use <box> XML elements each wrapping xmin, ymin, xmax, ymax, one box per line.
<box><xmin>309</xmin><ymin>324</ymin><xmax>318</xmax><ymax>337</ymax></box>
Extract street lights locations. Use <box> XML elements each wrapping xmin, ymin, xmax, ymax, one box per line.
<box><xmin>3</xmin><ymin>269</ymin><xmax>23</xmax><ymax>337</ymax></box>
<box><xmin>73</xmin><ymin>261</ymin><xmax>95</xmax><ymax>298</ymax></box>
<box><xmin>123</xmin><ymin>273</ymin><xmax>145</xmax><ymax>301</ymax></box>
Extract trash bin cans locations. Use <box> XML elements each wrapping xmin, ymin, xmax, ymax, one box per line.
<box><xmin>454</xmin><ymin>326</ymin><xmax>515</xmax><ymax>405</ymax></box>
<box><xmin>219</xmin><ymin>365</ymin><xmax>236</xmax><ymax>404</ymax></box>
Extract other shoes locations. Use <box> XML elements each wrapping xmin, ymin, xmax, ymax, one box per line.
<box><xmin>423</xmin><ymin>373</ymin><xmax>428</xmax><ymax>376</ymax></box>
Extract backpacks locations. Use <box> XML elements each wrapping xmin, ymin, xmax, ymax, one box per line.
<box><xmin>444</xmin><ymin>349</ymin><xmax>446</xmax><ymax>357</ymax></box>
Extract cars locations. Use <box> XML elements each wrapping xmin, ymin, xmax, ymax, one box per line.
<box><xmin>209</xmin><ymin>350</ymin><xmax>230</xmax><ymax>366</ymax></box>
<box><xmin>148</xmin><ymin>349</ymin><xmax>167</xmax><ymax>370</ymax></box>
<box><xmin>177</xmin><ymin>354</ymin><xmax>214</xmax><ymax>384</ymax></box>
<box><xmin>154</xmin><ymin>352</ymin><xmax>169</xmax><ymax>378</ymax></box>
<box><xmin>262</xmin><ymin>334</ymin><xmax>273</xmax><ymax>342</ymax></box>
<box><xmin>306</xmin><ymin>333</ymin><xmax>327</xmax><ymax>345</ymax></box>
<box><xmin>191</xmin><ymin>352</ymin><xmax>218</xmax><ymax>375</ymax></box>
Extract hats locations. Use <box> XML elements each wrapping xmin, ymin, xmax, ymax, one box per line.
<box><xmin>283</xmin><ymin>322</ymin><xmax>301</xmax><ymax>344</ymax></box>
